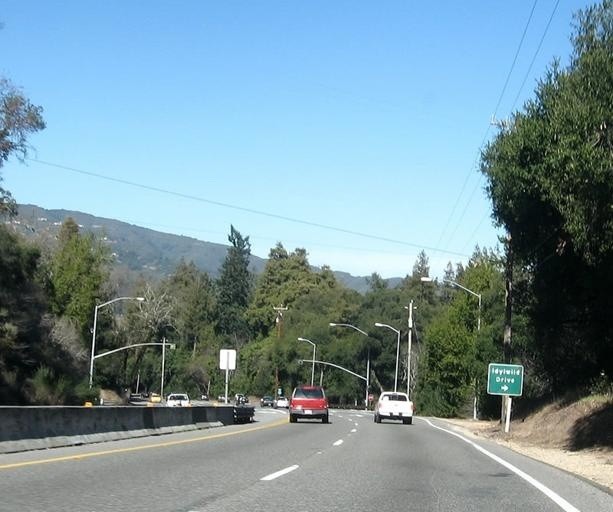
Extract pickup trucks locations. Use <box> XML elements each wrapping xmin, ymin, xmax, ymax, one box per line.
<box><xmin>374</xmin><ymin>391</ymin><xmax>415</xmax><ymax>426</ymax></box>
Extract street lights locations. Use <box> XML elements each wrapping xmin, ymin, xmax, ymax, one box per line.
<box><xmin>374</xmin><ymin>322</ymin><xmax>402</xmax><ymax>391</ymax></box>
<box><xmin>420</xmin><ymin>276</ymin><xmax>483</xmax><ymax>419</ymax></box>
<box><xmin>328</xmin><ymin>322</ymin><xmax>371</xmax><ymax>411</ymax></box>
<box><xmin>297</xmin><ymin>337</ymin><xmax>316</xmax><ymax>385</ymax></box>
<box><xmin>83</xmin><ymin>291</ymin><xmax>177</xmax><ymax>407</ymax></box>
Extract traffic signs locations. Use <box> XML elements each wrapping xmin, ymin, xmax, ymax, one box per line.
<box><xmin>486</xmin><ymin>363</ymin><xmax>524</xmax><ymax>397</ymax></box>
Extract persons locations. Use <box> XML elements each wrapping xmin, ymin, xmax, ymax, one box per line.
<box><xmin>238</xmin><ymin>394</ymin><xmax>248</xmax><ymax>404</ymax></box>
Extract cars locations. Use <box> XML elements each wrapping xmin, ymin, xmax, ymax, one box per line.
<box><xmin>217</xmin><ymin>386</ymin><xmax>290</xmax><ymax>408</ymax></box>
<box><xmin>165</xmin><ymin>393</ymin><xmax>190</xmax><ymax>411</ymax></box>
<box><xmin>289</xmin><ymin>384</ymin><xmax>328</xmax><ymax>425</ymax></box>
